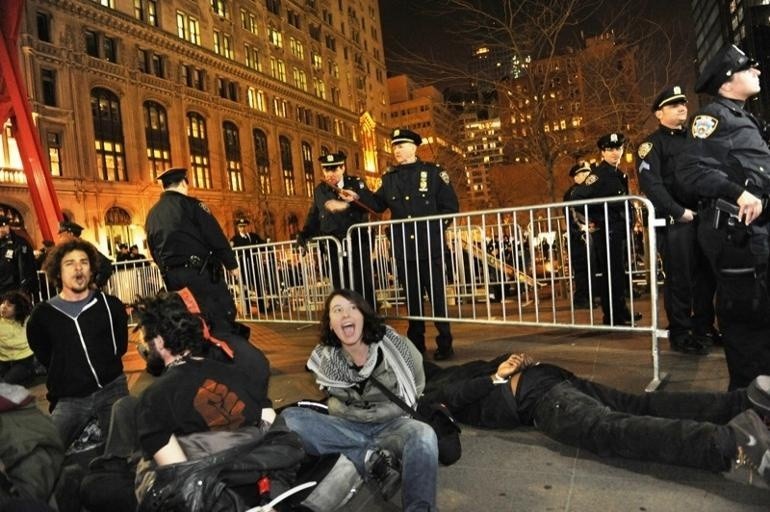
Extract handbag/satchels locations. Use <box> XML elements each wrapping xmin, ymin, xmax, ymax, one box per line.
<box><xmin>415</xmin><ymin>394</ymin><xmax>464</xmax><ymax>467</ymax></box>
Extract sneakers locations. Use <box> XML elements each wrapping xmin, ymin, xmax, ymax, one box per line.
<box><xmin>573</xmin><ymin>299</ymin><xmax>600</xmax><ymax>310</ymax></box>
<box><xmin>744</xmin><ymin>375</ymin><xmax>770</xmax><ymax>416</ymax></box>
<box><xmin>603</xmin><ymin>319</ymin><xmax>636</xmax><ymax>332</ymax></box>
<box><xmin>670</xmin><ymin>333</ymin><xmax>710</xmax><ymax>355</ymax></box>
<box><xmin>721</xmin><ymin>408</ymin><xmax>770</xmax><ymax>489</ymax></box>
<box><xmin>433</xmin><ymin>340</ymin><xmax>455</xmax><ymax>361</ymax></box>
<box><xmin>360</xmin><ymin>447</ymin><xmax>403</xmax><ymax>499</ymax></box>
<box><xmin>620</xmin><ymin>310</ymin><xmax>643</xmax><ymax>318</ymax></box>
<box><xmin>694</xmin><ymin>324</ymin><xmax>728</xmax><ymax>346</ymax></box>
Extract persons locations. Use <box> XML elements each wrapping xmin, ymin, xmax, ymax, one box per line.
<box><xmin>297</xmin><ymin>154</ymin><xmax>371</xmax><ymax>318</ymax></box>
<box><xmin>88</xmin><ymin>289</ymin><xmax>273</xmax><ymax>467</ymax></box>
<box><xmin>128</xmin><ymin>246</ymin><xmax>151</xmax><ymax>268</ymax></box>
<box><xmin>422</xmin><ymin>355</ymin><xmax>769</xmax><ymax>486</ymax></box>
<box><xmin>144</xmin><ymin>170</ymin><xmax>241</xmax><ymax>328</ymax></box>
<box><xmin>1</xmin><ymin>293</ymin><xmax>36</xmax><ymax>386</ymax></box>
<box><xmin>231</xmin><ymin>220</ymin><xmax>266</xmax><ymax>253</ymax></box>
<box><xmin>57</xmin><ymin>219</ymin><xmax>112</xmax><ymax>291</ymax></box>
<box><xmin>571</xmin><ymin>132</ymin><xmax>641</xmax><ymax>325</ymax></box>
<box><xmin>278</xmin><ymin>289</ymin><xmax>438</xmax><ymax>512</ymax></box>
<box><xmin>116</xmin><ymin>244</ymin><xmax>133</xmax><ymax>269</ymax></box>
<box><xmin>561</xmin><ymin>161</ymin><xmax>603</xmax><ymax>309</ymax></box>
<box><xmin>636</xmin><ymin>85</ymin><xmax>726</xmax><ymax>353</ymax></box>
<box><xmin>445</xmin><ymin>230</ymin><xmax>549</xmax><ymax>305</ymax></box>
<box><xmin>339</xmin><ymin>127</ymin><xmax>458</xmax><ymax>358</ymax></box>
<box><xmin>674</xmin><ymin>42</ymin><xmax>770</xmax><ymax>388</ymax></box>
<box><xmin>25</xmin><ymin>239</ymin><xmax>131</xmax><ymax>440</ymax></box>
<box><xmin>1</xmin><ymin>218</ymin><xmax>37</xmax><ymax>297</ymax></box>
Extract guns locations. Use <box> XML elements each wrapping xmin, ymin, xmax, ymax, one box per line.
<box><xmin>715</xmin><ymin>197</ymin><xmax>744</xmax><ymax>232</ymax></box>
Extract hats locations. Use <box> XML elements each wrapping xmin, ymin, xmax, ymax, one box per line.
<box><xmin>58</xmin><ymin>222</ymin><xmax>84</xmax><ymax>237</ymax></box>
<box><xmin>693</xmin><ymin>39</ymin><xmax>753</xmax><ymax>94</ymax></box>
<box><xmin>389</xmin><ymin>129</ymin><xmax>422</xmax><ymax>146</ymax></box>
<box><xmin>0</xmin><ymin>215</ymin><xmax>11</xmax><ymax>227</ymax></box>
<box><xmin>569</xmin><ymin>160</ymin><xmax>592</xmax><ymax>176</ymax></box>
<box><xmin>597</xmin><ymin>132</ymin><xmax>626</xmax><ymax>149</ymax></box>
<box><xmin>157</xmin><ymin>168</ymin><xmax>187</xmax><ymax>183</ymax></box>
<box><xmin>651</xmin><ymin>84</ymin><xmax>689</xmax><ymax>112</ymax></box>
<box><xmin>318</xmin><ymin>154</ymin><xmax>347</xmax><ymax>167</ymax></box>
